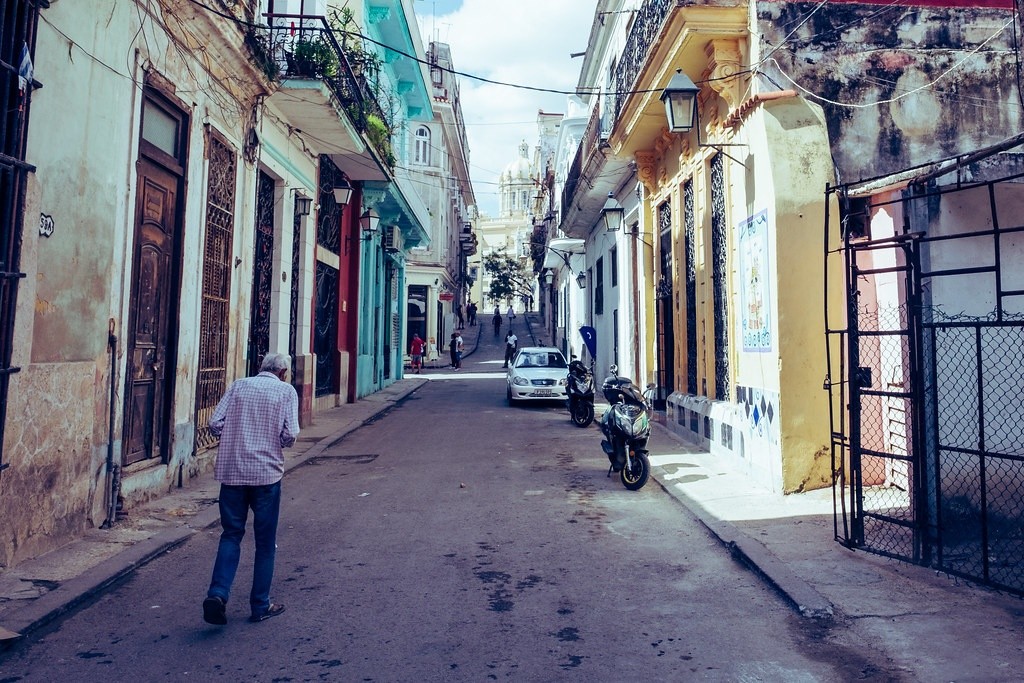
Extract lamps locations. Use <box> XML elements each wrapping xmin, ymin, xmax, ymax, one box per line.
<box><xmin>600</xmin><ymin>191</ymin><xmax>653</xmax><ymax>247</ymax></box>
<box><xmin>660</xmin><ymin>67</ymin><xmax>752</xmax><ymax>172</ymax></box>
<box><xmin>545</xmin><ymin>270</ymin><xmax>554</xmax><ymax>284</ymax></box>
<box><xmin>316</xmin><ymin>175</ymin><xmax>354</xmax><ymax>207</ymax></box>
<box><xmin>345</xmin><ymin>207</ymin><xmax>381</xmax><ymax>256</ymax></box>
<box><xmin>289</xmin><ymin>188</ymin><xmax>313</xmax><ymax>215</ymax></box>
<box><xmin>575</xmin><ymin>272</ymin><xmax>586</xmax><ymax>288</ymax></box>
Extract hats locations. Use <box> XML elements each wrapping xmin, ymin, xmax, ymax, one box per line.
<box><xmin>414</xmin><ymin>334</ymin><xmax>418</xmax><ymax>337</ymax></box>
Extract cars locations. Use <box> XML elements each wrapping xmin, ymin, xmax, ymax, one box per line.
<box><xmin>506</xmin><ymin>346</ymin><xmax>570</xmax><ymax>406</ymax></box>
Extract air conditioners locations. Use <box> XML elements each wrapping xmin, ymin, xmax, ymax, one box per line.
<box><xmin>385</xmin><ymin>225</ymin><xmax>402</xmax><ymax>252</ymax></box>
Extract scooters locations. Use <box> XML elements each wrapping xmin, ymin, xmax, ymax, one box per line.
<box><xmin>600</xmin><ymin>364</ymin><xmax>658</xmax><ymax>489</ymax></box>
<box><xmin>566</xmin><ymin>355</ymin><xmax>596</xmax><ymax>427</ymax></box>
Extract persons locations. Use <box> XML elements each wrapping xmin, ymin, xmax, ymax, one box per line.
<box><xmin>502</xmin><ymin>329</ymin><xmax>517</xmax><ymax>368</ymax></box>
<box><xmin>456</xmin><ymin>305</ymin><xmax>465</xmax><ymax>330</ymax></box>
<box><xmin>410</xmin><ymin>334</ymin><xmax>426</xmax><ymax>374</ymax></box>
<box><xmin>507</xmin><ymin>305</ymin><xmax>515</xmax><ymax>327</ymax></box>
<box><xmin>449</xmin><ymin>332</ymin><xmax>463</xmax><ymax>371</ymax></box>
<box><xmin>492</xmin><ymin>312</ymin><xmax>502</xmax><ymax>337</ymax></box>
<box><xmin>494</xmin><ymin>305</ymin><xmax>500</xmax><ymax>314</ymax></box>
<box><xmin>466</xmin><ymin>303</ymin><xmax>477</xmax><ymax>327</ymax></box>
<box><xmin>202</xmin><ymin>354</ymin><xmax>300</xmax><ymax>624</ymax></box>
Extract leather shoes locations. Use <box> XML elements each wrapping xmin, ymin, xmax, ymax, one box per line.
<box><xmin>203</xmin><ymin>596</ymin><xmax>227</xmax><ymax>625</ymax></box>
<box><xmin>251</xmin><ymin>603</ymin><xmax>285</xmax><ymax>621</ymax></box>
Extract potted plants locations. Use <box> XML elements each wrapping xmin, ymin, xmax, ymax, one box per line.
<box><xmin>286</xmin><ymin>35</ymin><xmax>335</xmax><ymax>78</ymax></box>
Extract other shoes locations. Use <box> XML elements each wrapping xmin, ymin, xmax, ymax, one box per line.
<box><xmin>416</xmin><ymin>370</ymin><xmax>420</xmax><ymax>373</ymax></box>
<box><xmin>455</xmin><ymin>367</ymin><xmax>460</xmax><ymax>371</ymax></box>
<box><xmin>502</xmin><ymin>366</ymin><xmax>508</xmax><ymax>368</ymax></box>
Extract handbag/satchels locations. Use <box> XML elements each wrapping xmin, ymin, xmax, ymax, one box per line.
<box><xmin>507</xmin><ymin>343</ymin><xmax>512</xmax><ymax>348</ymax></box>
